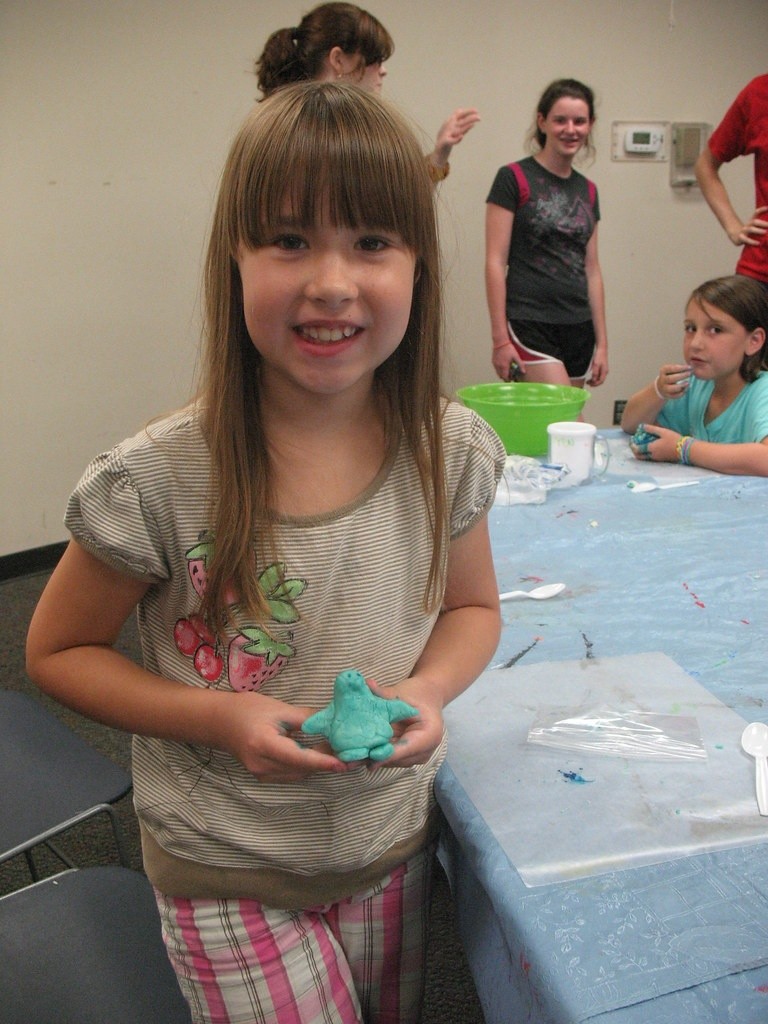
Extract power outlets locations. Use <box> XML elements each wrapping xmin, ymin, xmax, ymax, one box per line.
<box><xmin>613</xmin><ymin>400</ymin><xmax>628</xmax><ymax>425</ymax></box>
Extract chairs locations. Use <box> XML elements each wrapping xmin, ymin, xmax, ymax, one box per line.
<box><xmin>0</xmin><ymin>863</ymin><xmax>192</xmax><ymax>1024</ymax></box>
<box><xmin>0</xmin><ymin>689</ymin><xmax>133</xmax><ymax>882</ymax></box>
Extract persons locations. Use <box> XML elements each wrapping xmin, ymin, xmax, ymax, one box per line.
<box><xmin>484</xmin><ymin>78</ymin><xmax>608</xmax><ymax>424</ymax></box>
<box><xmin>696</xmin><ymin>72</ymin><xmax>768</xmax><ymax>285</ymax></box>
<box><xmin>24</xmin><ymin>85</ymin><xmax>508</xmax><ymax>1023</ymax></box>
<box><xmin>253</xmin><ymin>3</ymin><xmax>481</xmax><ymax>187</ymax></box>
<box><xmin>621</xmin><ymin>274</ymin><xmax>768</xmax><ymax>477</ymax></box>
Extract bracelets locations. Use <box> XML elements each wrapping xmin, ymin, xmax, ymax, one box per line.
<box><xmin>676</xmin><ymin>435</ymin><xmax>696</xmax><ymax>466</ymax></box>
<box><xmin>654</xmin><ymin>375</ymin><xmax>670</xmax><ymax>400</ymax></box>
<box><xmin>493</xmin><ymin>341</ymin><xmax>512</xmax><ymax>350</ymax></box>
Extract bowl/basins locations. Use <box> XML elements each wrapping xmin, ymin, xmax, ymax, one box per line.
<box><xmin>455</xmin><ymin>382</ymin><xmax>592</xmax><ymax>459</ymax></box>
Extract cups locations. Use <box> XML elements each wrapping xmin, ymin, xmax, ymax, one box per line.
<box><xmin>545</xmin><ymin>421</ymin><xmax>609</xmax><ymax>485</ymax></box>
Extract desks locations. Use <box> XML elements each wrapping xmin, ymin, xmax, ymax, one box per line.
<box><xmin>434</xmin><ymin>427</ymin><xmax>768</xmax><ymax>1024</ymax></box>
